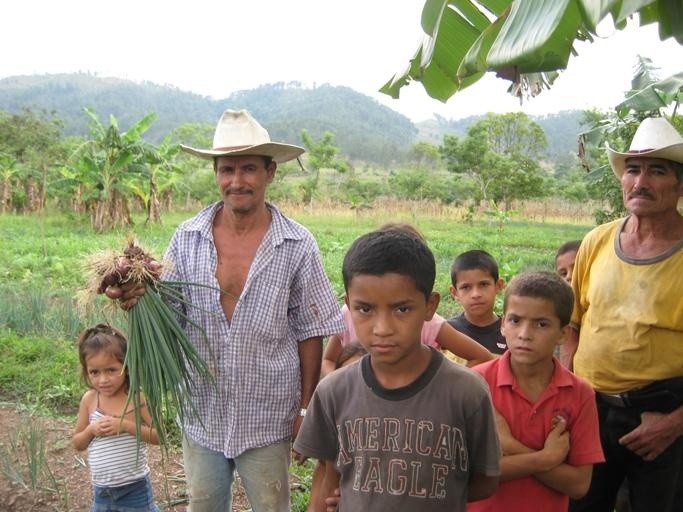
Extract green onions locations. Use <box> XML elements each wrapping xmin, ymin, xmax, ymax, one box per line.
<box><xmin>75</xmin><ymin>233</ymin><xmax>243</xmax><ymax>468</ymax></box>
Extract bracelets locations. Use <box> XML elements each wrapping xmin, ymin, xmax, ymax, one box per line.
<box><xmin>300</xmin><ymin>405</ymin><xmax>313</xmax><ymax>418</ymax></box>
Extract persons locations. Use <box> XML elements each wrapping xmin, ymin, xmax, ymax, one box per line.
<box><xmin>461</xmin><ymin>269</ymin><xmax>607</xmax><ymax>512</ymax></box>
<box><xmin>106</xmin><ymin>104</ymin><xmax>347</xmax><ymax>512</ymax></box>
<box><xmin>556</xmin><ymin>117</ymin><xmax>683</xmax><ymax>512</ymax></box>
<box><xmin>552</xmin><ymin>241</ymin><xmax>585</xmax><ymax>283</ymax></box>
<box><xmin>444</xmin><ymin>249</ymin><xmax>509</xmax><ymax>359</ymax></box>
<box><xmin>70</xmin><ymin>321</ymin><xmax>167</xmax><ymax>511</ymax></box>
<box><xmin>318</xmin><ymin>300</ymin><xmax>497</xmax><ymax>377</ymax></box>
<box><xmin>291</xmin><ymin>219</ymin><xmax>502</xmax><ymax>512</ymax></box>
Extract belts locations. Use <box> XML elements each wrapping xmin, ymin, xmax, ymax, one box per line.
<box><xmin>597</xmin><ymin>383</ymin><xmax>683</xmax><ymax>408</ymax></box>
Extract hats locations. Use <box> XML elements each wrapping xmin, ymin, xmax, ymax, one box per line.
<box><xmin>178</xmin><ymin>107</ymin><xmax>305</xmax><ymax>164</ymax></box>
<box><xmin>604</xmin><ymin>115</ymin><xmax>682</xmax><ymax>182</ymax></box>
